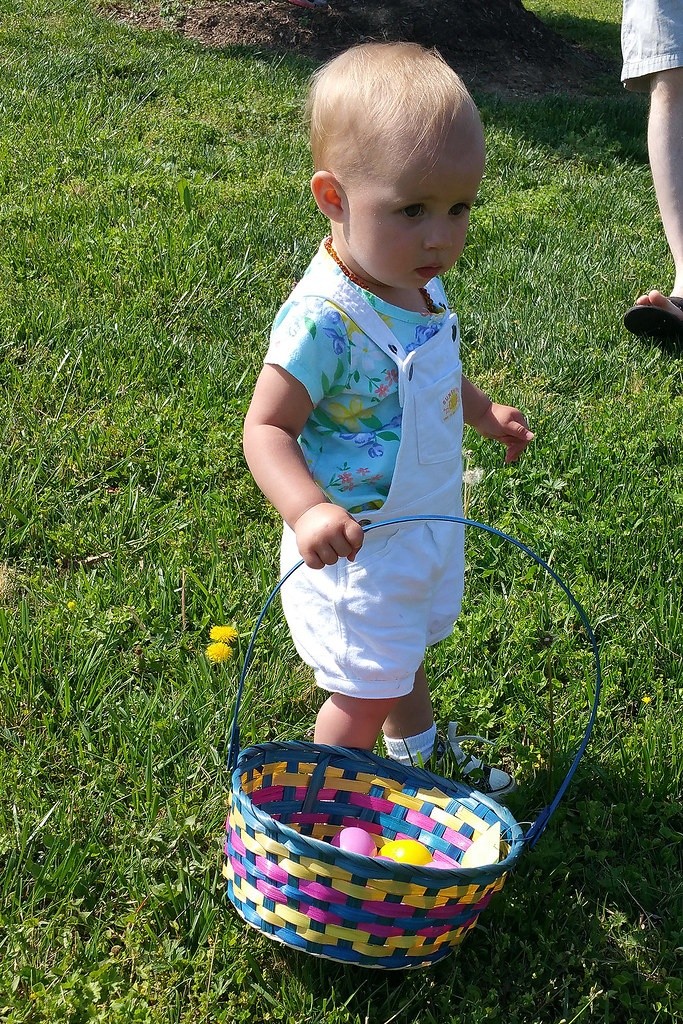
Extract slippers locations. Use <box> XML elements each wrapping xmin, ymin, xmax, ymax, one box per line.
<box><xmin>624</xmin><ymin>292</ymin><xmax>683</xmax><ymax>352</ymax></box>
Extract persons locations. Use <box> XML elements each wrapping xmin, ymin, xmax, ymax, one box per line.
<box><xmin>243</xmin><ymin>41</ymin><xmax>534</xmax><ymax>801</ymax></box>
<box><xmin>619</xmin><ymin>0</ymin><xmax>683</xmax><ymax>348</ymax></box>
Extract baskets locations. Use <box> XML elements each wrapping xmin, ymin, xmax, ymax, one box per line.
<box><xmin>217</xmin><ymin>511</ymin><xmax>604</xmax><ymax>973</ymax></box>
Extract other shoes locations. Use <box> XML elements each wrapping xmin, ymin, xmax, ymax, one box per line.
<box><xmin>400</xmin><ymin>720</ymin><xmax>516</xmax><ymax>799</ymax></box>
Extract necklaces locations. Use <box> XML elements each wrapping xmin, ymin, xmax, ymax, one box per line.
<box><xmin>323</xmin><ymin>236</ymin><xmax>437</xmax><ymax>316</ymax></box>
